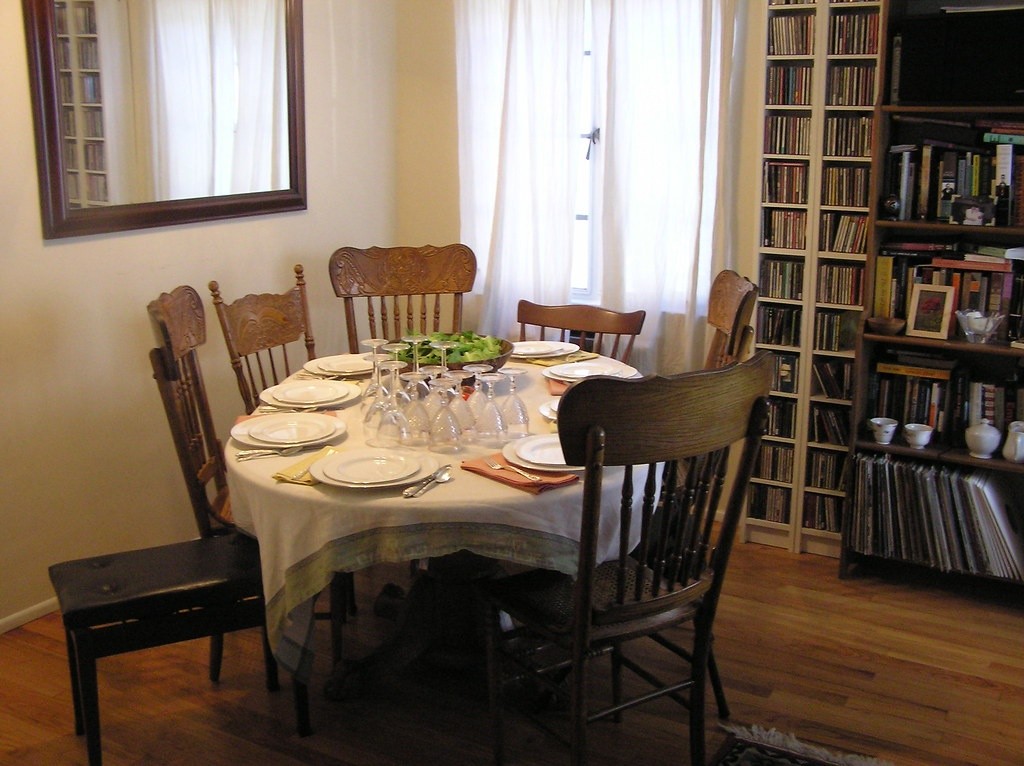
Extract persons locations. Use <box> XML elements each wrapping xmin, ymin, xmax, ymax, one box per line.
<box><xmin>942</xmin><ymin>184</ymin><xmax>955</xmax><ymax>199</ymax></box>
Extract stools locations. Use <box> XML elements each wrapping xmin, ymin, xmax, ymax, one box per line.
<box><xmin>50</xmin><ymin>532</ymin><xmax>314</xmax><ymax>766</ymax></box>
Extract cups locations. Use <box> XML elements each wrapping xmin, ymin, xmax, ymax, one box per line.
<box><xmin>965</xmin><ymin>418</ymin><xmax>1002</xmax><ymax>459</ymax></box>
<box><xmin>903</xmin><ymin>423</ymin><xmax>934</xmax><ymax>449</ymax></box>
<box><xmin>869</xmin><ymin>417</ymin><xmax>898</xmax><ymax>445</ymax></box>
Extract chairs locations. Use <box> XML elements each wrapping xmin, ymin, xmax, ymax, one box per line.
<box><xmin>145</xmin><ymin>246</ymin><xmax>779</xmax><ymax>765</ymax></box>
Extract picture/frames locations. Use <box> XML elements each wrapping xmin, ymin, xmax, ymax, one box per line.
<box><xmin>906</xmin><ymin>284</ymin><xmax>956</xmax><ymax>340</ymax></box>
<box><xmin>949</xmin><ymin>194</ymin><xmax>998</xmax><ymax>226</ymax></box>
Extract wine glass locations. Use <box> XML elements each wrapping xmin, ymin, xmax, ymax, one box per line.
<box><xmin>359</xmin><ymin>333</ymin><xmax>529</xmax><ymax>454</ymax></box>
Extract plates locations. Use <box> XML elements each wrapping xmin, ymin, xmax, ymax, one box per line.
<box><xmin>272</xmin><ymin>380</ymin><xmax>349</xmax><ymax>404</ymax></box>
<box><xmin>550</xmin><ymin>398</ymin><xmax>559</xmax><ymax>412</ymax></box>
<box><xmin>541</xmin><ymin>361</ymin><xmax>638</xmax><ymax>383</ymax></box>
<box><xmin>230</xmin><ymin>412</ymin><xmax>346</xmax><ymax>449</ymax></box>
<box><xmin>502</xmin><ymin>433</ymin><xmax>585</xmax><ymax>471</ymax></box>
<box><xmin>538</xmin><ymin>399</ymin><xmax>559</xmax><ymax>419</ymax></box>
<box><xmin>259</xmin><ymin>381</ymin><xmax>361</xmax><ymax>409</ymax></box>
<box><xmin>318</xmin><ymin>354</ymin><xmax>373</xmax><ymax>372</ymax></box>
<box><xmin>515</xmin><ymin>435</ymin><xmax>567</xmax><ymax>468</ymax></box>
<box><xmin>549</xmin><ymin>362</ymin><xmax>621</xmax><ymax>379</ymax></box>
<box><xmin>309</xmin><ymin>447</ymin><xmax>439</xmax><ymax>489</ymax></box>
<box><xmin>511</xmin><ymin>341</ymin><xmax>563</xmax><ymax>355</ymax></box>
<box><xmin>248</xmin><ymin>414</ymin><xmax>336</xmax><ymax>444</ymax></box>
<box><xmin>510</xmin><ymin>341</ymin><xmax>580</xmax><ymax>359</ymax></box>
<box><xmin>322</xmin><ymin>448</ymin><xmax>421</xmax><ymax>484</ymax></box>
<box><xmin>303</xmin><ymin>353</ymin><xmax>392</xmax><ymax>376</ymax></box>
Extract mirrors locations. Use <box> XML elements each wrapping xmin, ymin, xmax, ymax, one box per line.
<box><xmin>20</xmin><ymin>0</ymin><xmax>307</xmax><ymax>240</ymax></box>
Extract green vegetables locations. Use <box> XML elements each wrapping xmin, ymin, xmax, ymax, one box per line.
<box><xmin>389</xmin><ymin>327</ymin><xmax>502</xmax><ymax>365</ymax></box>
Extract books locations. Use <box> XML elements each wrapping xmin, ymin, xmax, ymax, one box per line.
<box><xmin>761</xmin><ymin>259</ymin><xmax>864</xmax><ymax>305</ymax></box>
<box><xmin>765</xmin><ymin>115</ymin><xmax>872</xmax><ymax>157</ymax></box>
<box><xmin>760</xmin><ymin>443</ymin><xmax>848</xmax><ymax>491</ymax></box>
<box><xmin>767</xmin><ymin>403</ymin><xmax>846</xmax><ymax>446</ymax></box>
<box><xmin>765</xmin><ymin>210</ymin><xmax>869</xmax><ymax>254</ymax></box>
<box><xmin>746</xmin><ymin>484</ymin><xmax>839</xmax><ymax>532</ymax></box>
<box><xmin>762</xmin><ymin>162</ymin><xmax>871</xmax><ymax>207</ymax></box>
<box><xmin>769</xmin><ymin>0</ymin><xmax>880</xmax><ymax>55</ymax></box>
<box><xmin>874</xmin><ymin>243</ymin><xmax>1024</xmax><ymax>341</ymax></box>
<box><xmin>886</xmin><ymin>115</ymin><xmax>1024</xmax><ymax>225</ymax></box>
<box><xmin>55</xmin><ymin>0</ymin><xmax>107</xmax><ymax>208</ymax></box>
<box><xmin>870</xmin><ymin>347</ymin><xmax>1024</xmax><ymax>448</ymax></box>
<box><xmin>767</xmin><ymin>65</ymin><xmax>875</xmax><ymax>106</ymax></box>
<box><xmin>757</xmin><ymin>306</ymin><xmax>841</xmax><ymax>351</ymax></box>
<box><xmin>772</xmin><ymin>356</ymin><xmax>852</xmax><ymax>400</ymax></box>
<box><xmin>889</xmin><ymin>32</ymin><xmax>928</xmax><ymax>105</ymax></box>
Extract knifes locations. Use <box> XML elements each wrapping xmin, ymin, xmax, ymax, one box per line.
<box><xmin>402</xmin><ymin>463</ymin><xmax>452</xmax><ymax>498</ymax></box>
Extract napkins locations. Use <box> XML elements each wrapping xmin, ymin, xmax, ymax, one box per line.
<box><xmin>234</xmin><ymin>411</ymin><xmax>338</xmax><ymax>426</ymax></box>
<box><xmin>272</xmin><ymin>446</ymin><xmax>349</xmax><ymax>485</ymax></box>
<box><xmin>526</xmin><ymin>350</ymin><xmax>599</xmax><ymax>366</ymax></box>
<box><xmin>297</xmin><ymin>368</ymin><xmax>362</xmax><ymax>385</ymax></box>
<box><xmin>460</xmin><ymin>453</ymin><xmax>579</xmax><ymax>495</ymax></box>
<box><xmin>547</xmin><ymin>378</ymin><xmax>571</xmax><ymax>396</ymax></box>
<box><xmin>550</xmin><ymin>420</ymin><xmax>558</xmax><ymax>433</ymax></box>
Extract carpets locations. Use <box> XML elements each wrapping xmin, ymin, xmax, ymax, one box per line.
<box><xmin>707</xmin><ymin>723</ymin><xmax>903</xmax><ymax>766</ymax></box>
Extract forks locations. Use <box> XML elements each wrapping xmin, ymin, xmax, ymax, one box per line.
<box><xmin>483</xmin><ymin>457</ymin><xmax>540</xmax><ymax>481</ymax></box>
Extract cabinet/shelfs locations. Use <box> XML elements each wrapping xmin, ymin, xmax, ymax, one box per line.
<box><xmin>51</xmin><ymin>0</ymin><xmax>134</xmax><ymax>209</ymax></box>
<box><xmin>739</xmin><ymin>0</ymin><xmax>1024</xmax><ymax>611</ymax></box>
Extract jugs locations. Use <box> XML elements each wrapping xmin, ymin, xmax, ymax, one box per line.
<box><xmin>1001</xmin><ymin>421</ymin><xmax>1024</xmax><ymax>464</ymax></box>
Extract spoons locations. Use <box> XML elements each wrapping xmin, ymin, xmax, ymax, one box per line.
<box><xmin>237</xmin><ymin>446</ymin><xmax>303</xmax><ymax>462</ymax></box>
<box><xmin>412</xmin><ymin>467</ymin><xmax>454</xmax><ymax>498</ymax></box>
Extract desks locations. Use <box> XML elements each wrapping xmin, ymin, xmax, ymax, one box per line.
<box><xmin>224</xmin><ymin>346</ymin><xmax>671</xmax><ymax>713</ymax></box>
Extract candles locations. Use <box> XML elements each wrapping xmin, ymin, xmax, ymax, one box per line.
<box><xmin>967</xmin><ymin>310</ymin><xmax>993</xmax><ymax>331</ymax></box>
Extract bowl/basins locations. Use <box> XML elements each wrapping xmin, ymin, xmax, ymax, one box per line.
<box><xmin>376</xmin><ymin>335</ymin><xmax>515</xmax><ymax>390</ymax></box>
